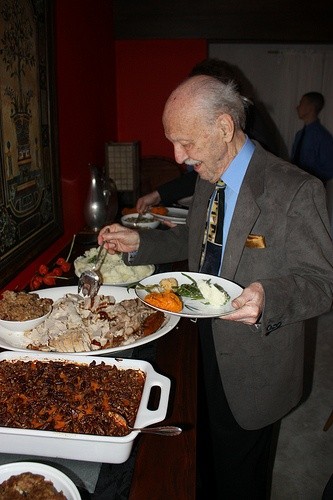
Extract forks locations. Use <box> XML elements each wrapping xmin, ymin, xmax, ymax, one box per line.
<box><xmin>78</xmin><ymin>228</ymin><xmax>109</xmax><ymax>299</ymax></box>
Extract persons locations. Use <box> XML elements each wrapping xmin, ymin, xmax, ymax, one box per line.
<box><xmin>97</xmin><ymin>74</ymin><xmax>333</xmax><ymax>500</ymax></box>
<box><xmin>136</xmin><ymin>59</ymin><xmax>333</xmax><ymax>215</ymax></box>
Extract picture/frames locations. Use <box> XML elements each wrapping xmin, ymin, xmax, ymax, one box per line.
<box><xmin>0</xmin><ymin>0</ymin><xmax>65</xmax><ymax>290</ymax></box>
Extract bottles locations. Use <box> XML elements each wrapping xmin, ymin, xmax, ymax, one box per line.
<box><xmin>85</xmin><ymin>161</ymin><xmax>108</xmax><ymax>231</ymax></box>
<box><xmin>100</xmin><ymin>162</ymin><xmax>118</xmax><ymax>228</ymax></box>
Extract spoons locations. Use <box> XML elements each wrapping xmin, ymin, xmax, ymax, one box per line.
<box><xmin>109</xmin><ymin>412</ymin><xmax>182</xmax><ymax>435</ymax></box>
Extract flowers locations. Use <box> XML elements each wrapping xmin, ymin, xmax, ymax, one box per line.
<box><xmin>30</xmin><ymin>258</ymin><xmax>71</xmax><ymax>290</ymax></box>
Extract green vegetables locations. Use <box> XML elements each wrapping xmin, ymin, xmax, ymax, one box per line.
<box><xmin>127</xmin><ymin>271</ymin><xmax>230</xmax><ymax>312</ymax></box>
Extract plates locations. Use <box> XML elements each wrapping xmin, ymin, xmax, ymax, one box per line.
<box><xmin>179</xmin><ymin>195</ymin><xmax>193</xmax><ymax>207</ymax></box>
<box><xmin>1</xmin><ymin>284</ymin><xmax>181</xmax><ymax>357</ymax></box>
<box><xmin>74</xmin><ymin>264</ymin><xmax>155</xmax><ymax>288</ymax></box>
<box><xmin>121</xmin><ymin>213</ymin><xmax>161</xmax><ymax>229</ymax></box>
<box><xmin>0</xmin><ymin>461</ymin><xmax>82</xmax><ymax>499</ymax></box>
<box><xmin>151</xmin><ymin>207</ymin><xmax>188</xmax><ymax>224</ymax></box>
<box><xmin>0</xmin><ymin>306</ymin><xmax>52</xmax><ymax>330</ymax></box>
<box><xmin>134</xmin><ymin>272</ymin><xmax>244</xmax><ymax>318</ymax></box>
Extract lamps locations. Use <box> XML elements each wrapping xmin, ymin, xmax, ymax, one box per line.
<box><xmin>105</xmin><ymin>141</ymin><xmax>141</xmax><ymax>207</ymax></box>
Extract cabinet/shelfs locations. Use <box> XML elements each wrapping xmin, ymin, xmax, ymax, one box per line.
<box><xmin>206</xmin><ymin>39</ymin><xmax>333</xmax><ymax>163</ymax></box>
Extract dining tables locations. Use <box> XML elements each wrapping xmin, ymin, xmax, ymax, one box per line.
<box><xmin>0</xmin><ymin>201</ymin><xmax>199</xmax><ymax>500</ymax></box>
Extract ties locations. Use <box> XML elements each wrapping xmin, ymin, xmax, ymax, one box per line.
<box><xmin>198</xmin><ymin>180</ymin><xmax>227</xmax><ymax>276</ymax></box>
